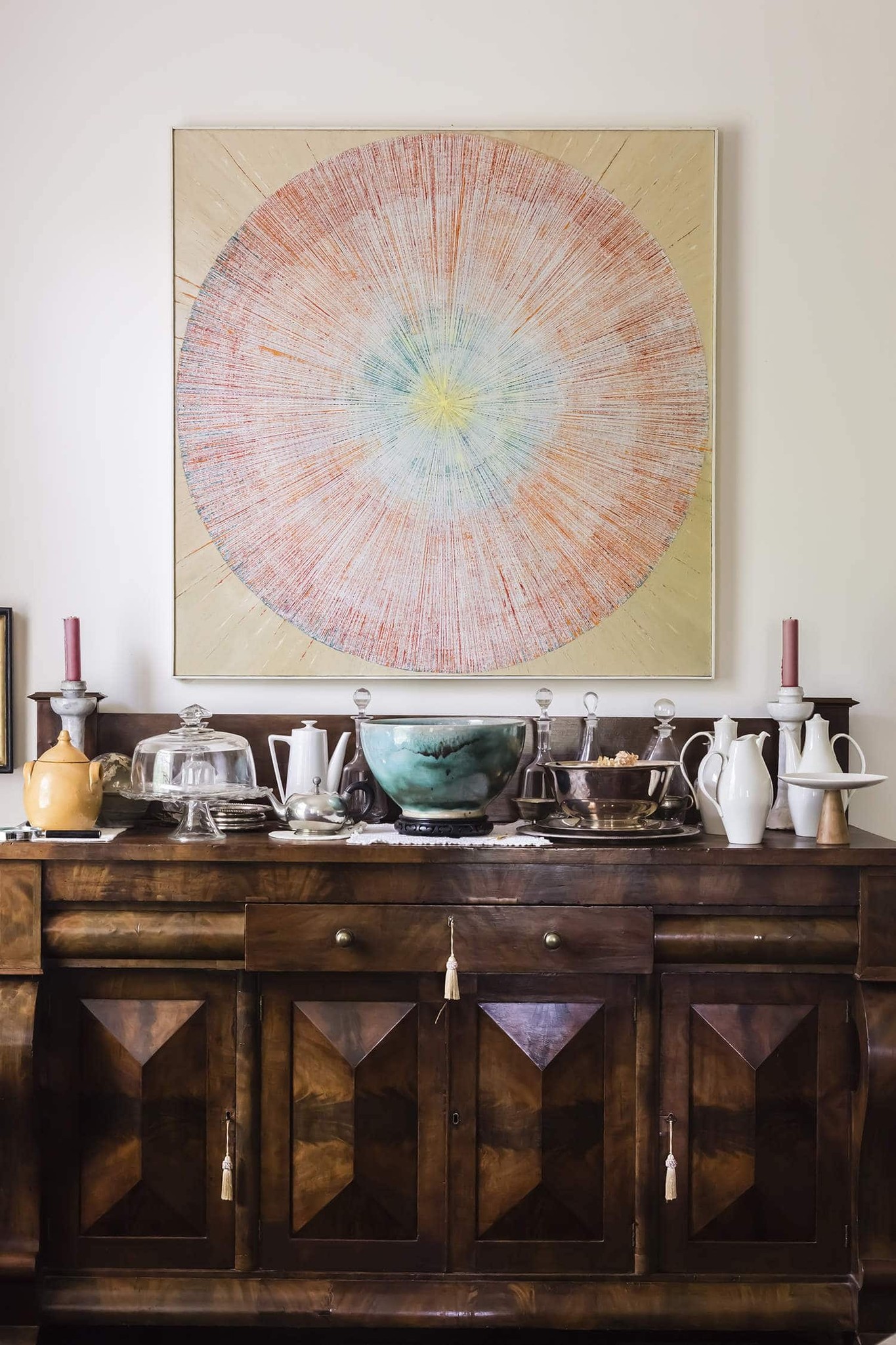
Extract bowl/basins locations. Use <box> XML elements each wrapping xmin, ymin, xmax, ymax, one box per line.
<box><xmin>652</xmin><ymin>795</ymin><xmax>693</xmax><ymax>819</ymax></box>
<box><xmin>360</xmin><ymin>717</ymin><xmax>526</xmax><ymax>838</ymax></box>
<box><xmin>536</xmin><ymin>760</ymin><xmax>682</xmax><ymax>828</ymax></box>
<box><xmin>512</xmin><ymin>797</ymin><xmax>557</xmax><ymax>824</ymax></box>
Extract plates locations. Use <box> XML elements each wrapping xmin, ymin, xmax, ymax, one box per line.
<box><xmin>516</xmin><ymin>818</ymin><xmax>700</xmax><ymax>839</ymax></box>
<box><xmin>777</xmin><ymin>773</ymin><xmax>888</xmax><ymax>789</ymax></box>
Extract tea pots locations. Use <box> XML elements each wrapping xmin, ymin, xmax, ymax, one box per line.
<box><xmin>268</xmin><ymin>720</ymin><xmax>352</xmax><ymax>803</ymax></box>
<box><xmin>267</xmin><ymin>776</ymin><xmax>375</xmax><ymax>835</ymax></box>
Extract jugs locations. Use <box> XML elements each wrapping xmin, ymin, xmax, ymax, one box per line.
<box><xmin>697</xmin><ymin>734</ymin><xmax>772</xmax><ymax>845</ymax></box>
<box><xmin>680</xmin><ymin>715</ymin><xmax>770</xmax><ymax>835</ymax></box>
<box><xmin>783</xmin><ymin>713</ymin><xmax>865</xmax><ymax>837</ymax></box>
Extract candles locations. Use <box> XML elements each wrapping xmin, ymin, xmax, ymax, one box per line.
<box><xmin>62</xmin><ymin>614</ymin><xmax>80</xmax><ymax>684</ymax></box>
<box><xmin>781</xmin><ymin>612</ymin><xmax>799</xmax><ymax>689</ymax></box>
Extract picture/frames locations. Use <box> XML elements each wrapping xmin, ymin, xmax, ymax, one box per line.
<box><xmin>0</xmin><ymin>606</ymin><xmax>13</xmax><ymax>775</ymax></box>
<box><xmin>169</xmin><ymin>127</ymin><xmax>718</xmax><ymax>680</ymax></box>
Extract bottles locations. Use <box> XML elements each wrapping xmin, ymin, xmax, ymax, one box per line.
<box><xmin>573</xmin><ymin>692</ymin><xmax>602</xmax><ymax>761</ymax></box>
<box><xmin>24</xmin><ymin>731</ymin><xmax>104</xmax><ymax>830</ymax></box>
<box><xmin>521</xmin><ymin>688</ymin><xmax>569</xmax><ymax>819</ymax></box>
<box><xmin>341</xmin><ymin>688</ymin><xmax>388</xmax><ymax>823</ymax></box>
<box><xmin>648</xmin><ymin>698</ymin><xmax>689</xmax><ymax>825</ymax></box>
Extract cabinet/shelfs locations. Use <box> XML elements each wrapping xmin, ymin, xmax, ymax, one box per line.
<box><xmin>0</xmin><ymin>825</ymin><xmax>896</xmax><ymax>1345</ymax></box>
